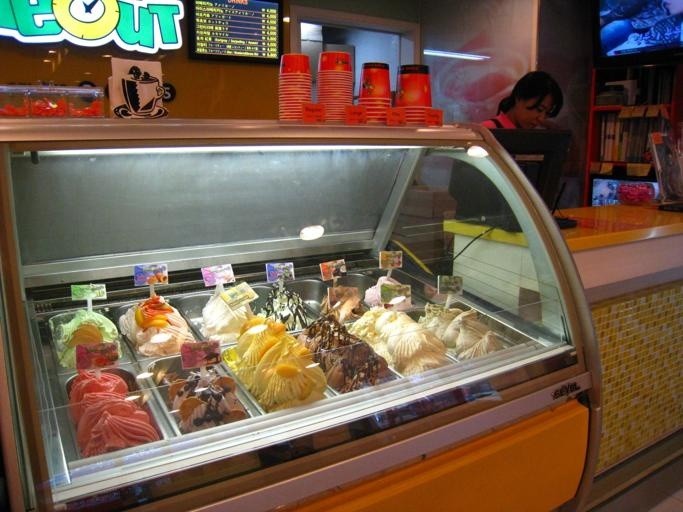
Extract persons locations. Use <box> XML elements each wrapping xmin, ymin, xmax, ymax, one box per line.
<box><xmin>481</xmin><ymin>71</ymin><xmax>564</xmax><ymax>129</ymax></box>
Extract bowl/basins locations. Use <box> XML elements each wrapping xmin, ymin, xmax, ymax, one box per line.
<box><xmin>320</xmin><ymin>272</ymin><xmax>426</xmax><ymax>309</ymax></box>
<box><xmin>250</xmin><ymin>286</ymin><xmax>315</xmax><ymax>333</ymax></box>
<box><xmin>342</xmin><ymin>321</ymin><xmax>458</xmax><ymax>378</ymax></box>
<box><xmin>278</xmin><ymin>54</ymin><xmax>312</xmax><ymax>124</ymax></box>
<box><xmin>290</xmin><ymin>332</ymin><xmax>400</xmax><ymax>396</ymax></box>
<box><xmin>57</xmin><ymin>363</ymin><xmax>175</xmax><ymax>460</ymax></box>
<box><xmin>317</xmin><ymin>52</ymin><xmax>353</xmax><ymax>122</ymax></box>
<box><xmin>173</xmin><ymin>293</ymin><xmax>239</xmax><ymax>347</ymax></box>
<box><xmin>110</xmin><ymin>300</ymin><xmax>201</xmax><ymax>362</ymax></box>
<box><xmin>138</xmin><ymin>354</ymin><xmax>262</xmax><ymax>436</ymax></box>
<box><xmin>272</xmin><ymin>278</ymin><xmax>370</xmax><ymax>323</ymax></box>
<box><xmin>44</xmin><ymin>309</ymin><xmax>136</xmax><ymax>376</ymax></box>
<box><xmin>402</xmin><ymin>309</ymin><xmax>519</xmax><ymax>363</ymax></box>
<box><xmin>219</xmin><ymin>343</ymin><xmax>337</xmax><ymax>416</ymax></box>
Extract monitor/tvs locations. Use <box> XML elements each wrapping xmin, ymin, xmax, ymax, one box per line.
<box><xmin>188</xmin><ymin>0</ymin><xmax>284</xmax><ymax>65</ymax></box>
<box><xmin>587</xmin><ymin>174</ymin><xmax>661</xmax><ymax>208</ymax></box>
<box><xmin>595</xmin><ymin>0</ymin><xmax>683</xmax><ymax>66</ymax></box>
<box><xmin>449</xmin><ymin>129</ymin><xmax>577</xmax><ymax>233</ymax></box>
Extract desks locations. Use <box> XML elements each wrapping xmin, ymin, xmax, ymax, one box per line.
<box><xmin>443</xmin><ymin>205</ymin><xmax>683</xmax><ymax>512</ymax></box>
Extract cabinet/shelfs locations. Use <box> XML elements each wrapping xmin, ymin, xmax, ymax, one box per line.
<box><xmin>0</xmin><ymin>117</ymin><xmax>605</xmax><ymax>511</ymax></box>
<box><xmin>584</xmin><ymin>57</ymin><xmax>683</xmax><ymax>213</ymax></box>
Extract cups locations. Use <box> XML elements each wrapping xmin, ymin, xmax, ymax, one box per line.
<box><xmin>358</xmin><ymin>63</ymin><xmax>392</xmax><ymax>123</ymax></box>
<box><xmin>392</xmin><ymin>64</ymin><xmax>432</xmax><ymax>127</ymax></box>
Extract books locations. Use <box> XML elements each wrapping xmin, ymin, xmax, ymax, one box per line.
<box><xmin>599</xmin><ymin>110</ymin><xmax>669</xmax><ymax>162</ymax></box>
<box><xmin>649</xmin><ymin>131</ymin><xmax>683</xmax><ymax>203</ymax></box>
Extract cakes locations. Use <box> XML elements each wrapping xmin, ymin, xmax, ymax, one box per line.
<box><xmin>202</xmin><ymin>288</ymin><xmax>254</xmax><ymax>346</ymax></box>
<box><xmin>262</xmin><ymin>283</ymin><xmax>317</xmax><ymax>333</ymax></box>
<box><xmin>117</xmin><ymin>294</ymin><xmax>197</xmax><ymax>359</ymax></box>
<box><xmin>50</xmin><ymin>310</ymin><xmax>121</xmax><ymax>369</ymax></box>
<box><xmin>297</xmin><ymin>311</ymin><xmax>395</xmax><ymax>395</ymax></box>
<box><xmin>319</xmin><ymin>285</ymin><xmax>368</xmax><ymax>326</ymax></box>
<box><xmin>347</xmin><ymin>303</ymin><xmax>449</xmax><ymax>378</ymax></box>
<box><xmin>221</xmin><ymin>314</ymin><xmax>327</xmax><ymax>415</ymax></box>
<box><xmin>417</xmin><ymin>302</ymin><xmax>513</xmax><ymax>364</ymax></box>
<box><xmin>363</xmin><ymin>275</ymin><xmax>414</xmax><ymax>312</ymax></box>
<box><xmin>67</xmin><ymin>370</ymin><xmax>160</xmax><ymax>458</ymax></box>
<box><xmin>169</xmin><ymin>369</ymin><xmax>249</xmax><ymax>436</ymax></box>
